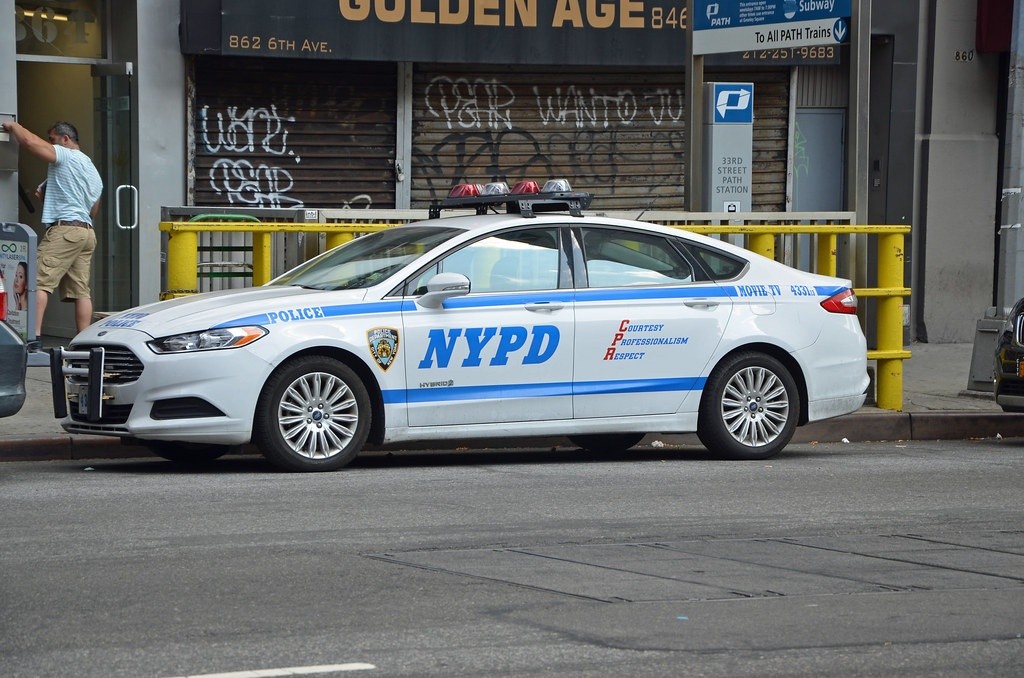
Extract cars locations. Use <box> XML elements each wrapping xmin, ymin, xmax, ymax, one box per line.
<box><xmin>0</xmin><ymin>272</ymin><xmax>28</xmax><ymax>419</ymax></box>
<box><xmin>993</xmin><ymin>297</ymin><xmax>1024</xmax><ymax>411</ymax></box>
<box><xmin>51</xmin><ymin>180</ymin><xmax>872</xmax><ymax>471</ymax></box>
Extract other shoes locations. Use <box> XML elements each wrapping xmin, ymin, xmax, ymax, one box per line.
<box><xmin>27</xmin><ymin>336</ymin><xmax>41</xmax><ymax>353</ymax></box>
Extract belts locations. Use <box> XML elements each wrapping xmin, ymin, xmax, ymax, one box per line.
<box><xmin>51</xmin><ymin>220</ymin><xmax>93</xmax><ymax>229</ymax></box>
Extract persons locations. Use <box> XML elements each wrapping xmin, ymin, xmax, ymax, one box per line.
<box><xmin>2</xmin><ymin>121</ymin><xmax>103</xmax><ymax>352</ymax></box>
<box><xmin>12</xmin><ymin>262</ymin><xmax>27</xmax><ymax>310</ymax></box>
